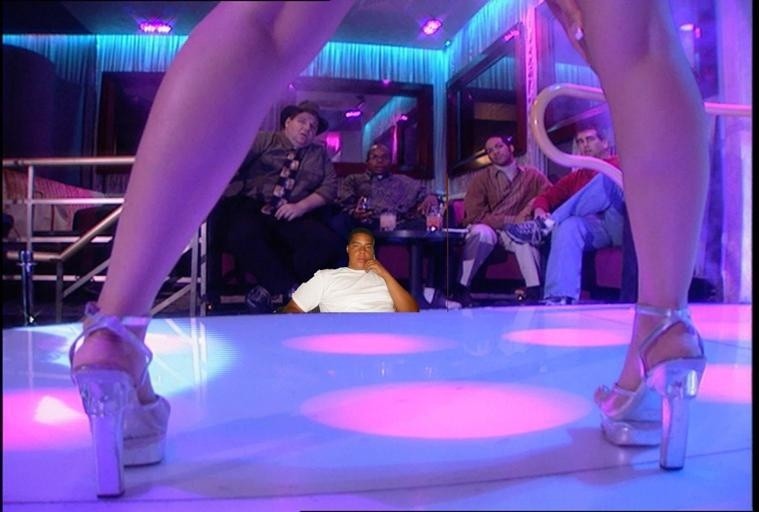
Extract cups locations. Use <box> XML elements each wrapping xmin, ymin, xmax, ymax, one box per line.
<box><xmin>379</xmin><ymin>211</ymin><xmax>398</xmax><ymax>232</ymax></box>
<box><xmin>427</xmin><ymin>211</ymin><xmax>443</xmax><ymax>232</ymax></box>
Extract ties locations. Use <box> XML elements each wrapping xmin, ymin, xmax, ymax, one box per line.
<box><xmin>267</xmin><ymin>148</ymin><xmax>302</xmax><ymax>213</ymax></box>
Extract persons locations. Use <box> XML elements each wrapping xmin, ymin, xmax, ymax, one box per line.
<box><xmin>68</xmin><ymin>0</ymin><xmax>717</xmax><ymax>500</ymax></box>
<box><xmin>282</xmin><ymin>226</ymin><xmax>420</xmax><ymax>312</ymax></box>
<box><xmin>336</xmin><ymin>140</ymin><xmax>461</xmax><ymax>309</ymax></box>
<box><xmin>501</xmin><ymin>122</ymin><xmax>625</xmax><ymax>304</ymax></box>
<box><xmin>355</xmin><ymin>95</ymin><xmax>369</xmax><ymax>112</ymax></box>
<box><xmin>452</xmin><ymin>132</ymin><xmax>553</xmax><ymax>306</ymax></box>
<box><xmin>203</xmin><ymin>98</ymin><xmax>337</xmax><ymax>311</ymax></box>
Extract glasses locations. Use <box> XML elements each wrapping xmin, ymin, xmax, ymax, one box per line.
<box><xmin>368</xmin><ymin>153</ymin><xmax>391</xmax><ymax>162</ymax></box>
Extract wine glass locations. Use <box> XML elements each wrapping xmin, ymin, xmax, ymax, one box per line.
<box><xmin>358</xmin><ymin>194</ymin><xmax>376</xmax><ymax>226</ymax></box>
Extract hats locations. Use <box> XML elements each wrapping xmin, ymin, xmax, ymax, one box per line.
<box><xmin>278</xmin><ymin>98</ymin><xmax>330</xmax><ymax>137</ymax></box>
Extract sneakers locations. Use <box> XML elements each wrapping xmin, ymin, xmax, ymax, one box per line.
<box><xmin>245</xmin><ymin>284</ymin><xmax>275</xmax><ymax>314</ymax></box>
<box><xmin>502</xmin><ymin>214</ymin><xmax>548</xmax><ymax>248</ymax></box>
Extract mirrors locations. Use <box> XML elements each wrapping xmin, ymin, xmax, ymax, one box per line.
<box><xmin>102</xmin><ymin>71</ymin><xmax>436</xmax><ymax>181</ymax></box>
<box><xmin>445</xmin><ymin>17</ymin><xmax>527</xmax><ymax>177</ymax></box>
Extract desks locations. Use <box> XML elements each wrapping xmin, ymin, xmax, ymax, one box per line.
<box><xmin>370</xmin><ymin>227</ymin><xmax>461</xmax><ymax>311</ymax></box>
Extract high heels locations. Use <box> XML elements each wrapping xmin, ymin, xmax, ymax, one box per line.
<box><xmin>66</xmin><ymin>299</ymin><xmax>173</xmax><ymax>501</ymax></box>
<box><xmin>598</xmin><ymin>300</ymin><xmax>709</xmax><ymax>473</ymax></box>
